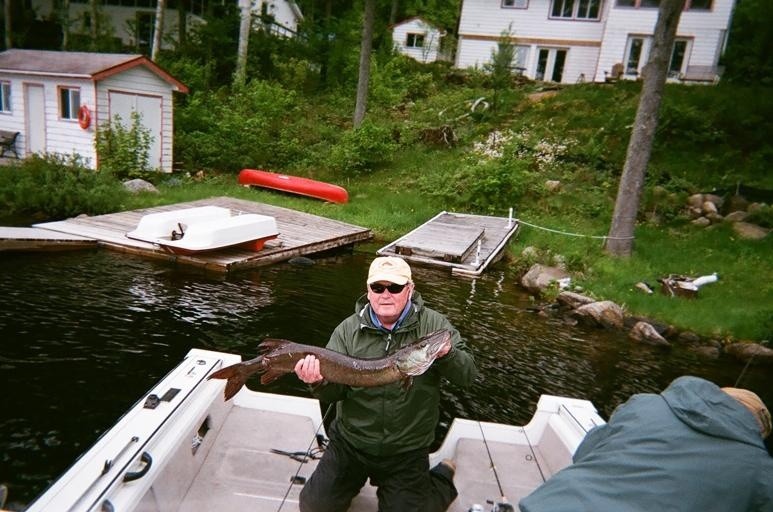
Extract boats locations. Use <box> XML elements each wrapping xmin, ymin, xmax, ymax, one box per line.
<box><xmin>124</xmin><ymin>205</ymin><xmax>280</xmax><ymax>256</ymax></box>
<box><xmin>21</xmin><ymin>348</ymin><xmax>607</xmax><ymax>511</ymax></box>
<box><xmin>237</xmin><ymin>169</ymin><xmax>349</xmax><ymax>204</ymax></box>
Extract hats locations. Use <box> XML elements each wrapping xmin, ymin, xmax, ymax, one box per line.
<box><xmin>366</xmin><ymin>256</ymin><xmax>413</xmax><ymax>286</ymax></box>
<box><xmin>721</xmin><ymin>387</ymin><xmax>772</xmax><ymax>438</ymax></box>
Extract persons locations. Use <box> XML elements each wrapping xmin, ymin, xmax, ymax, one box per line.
<box><xmin>292</xmin><ymin>252</ymin><xmax>482</xmax><ymax>511</ymax></box>
<box><xmin>518</xmin><ymin>373</ymin><xmax>772</xmax><ymax>512</ymax></box>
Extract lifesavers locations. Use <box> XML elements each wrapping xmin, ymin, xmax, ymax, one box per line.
<box><xmin>78</xmin><ymin>106</ymin><xmax>90</xmax><ymax>129</ymax></box>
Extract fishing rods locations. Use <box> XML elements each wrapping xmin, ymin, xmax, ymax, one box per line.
<box><xmin>466</xmin><ymin>395</ymin><xmax>547</xmax><ymax>512</ymax></box>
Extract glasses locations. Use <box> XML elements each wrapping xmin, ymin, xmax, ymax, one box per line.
<box><xmin>370</xmin><ymin>282</ymin><xmax>408</xmax><ymax>294</ymax></box>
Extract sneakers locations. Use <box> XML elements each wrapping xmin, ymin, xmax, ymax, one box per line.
<box><xmin>431</xmin><ymin>458</ymin><xmax>457</xmax><ymax>481</ymax></box>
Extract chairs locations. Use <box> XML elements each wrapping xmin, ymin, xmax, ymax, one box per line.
<box><xmin>604</xmin><ymin>64</ymin><xmax>623</xmax><ymax>82</ymax></box>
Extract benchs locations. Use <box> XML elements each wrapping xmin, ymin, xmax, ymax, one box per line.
<box><xmin>0</xmin><ymin>130</ymin><xmax>21</xmax><ymax>159</ymax></box>
<box><xmin>493</xmin><ymin>64</ymin><xmax>525</xmax><ymax>75</ymax></box>
<box><xmin>678</xmin><ymin>65</ymin><xmax>726</xmax><ymax>85</ymax></box>
